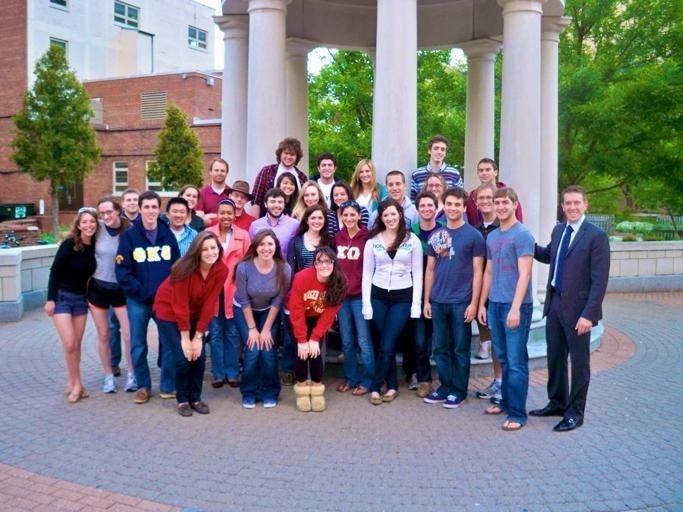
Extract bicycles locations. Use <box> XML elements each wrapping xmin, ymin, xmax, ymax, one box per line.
<box><xmin>0</xmin><ymin>229</ymin><xmax>48</xmax><ymax>250</ymax></box>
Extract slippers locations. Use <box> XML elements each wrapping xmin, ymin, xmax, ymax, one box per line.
<box><xmin>502</xmin><ymin>420</ymin><xmax>522</xmax><ymax>431</ymax></box>
<box><xmin>485</xmin><ymin>404</ymin><xmax>503</xmax><ymax>414</ymax></box>
<box><xmin>63</xmin><ymin>384</ymin><xmax>89</xmax><ymax>402</ymax></box>
<box><xmin>337</xmin><ymin>382</ymin><xmax>368</xmax><ymax>395</ymax></box>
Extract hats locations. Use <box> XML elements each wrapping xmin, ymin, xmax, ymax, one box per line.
<box><xmin>225</xmin><ymin>180</ymin><xmax>254</xmax><ymax>202</ymax></box>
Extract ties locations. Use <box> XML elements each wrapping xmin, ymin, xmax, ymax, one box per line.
<box><xmin>554</xmin><ymin>226</ymin><xmax>574</xmax><ymax>295</ymax></box>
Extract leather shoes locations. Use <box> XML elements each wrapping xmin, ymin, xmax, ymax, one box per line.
<box><xmin>554</xmin><ymin>413</ymin><xmax>584</xmax><ymax>431</ymax></box>
<box><xmin>528</xmin><ymin>401</ymin><xmax>563</xmax><ymax>416</ymax></box>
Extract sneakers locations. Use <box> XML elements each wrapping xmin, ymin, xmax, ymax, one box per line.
<box><xmin>408</xmin><ymin>379</ymin><xmax>506</xmax><ymax>408</ymax></box>
<box><xmin>159</xmin><ymin>378</ymin><xmax>278</xmax><ymax>418</ymax></box>
<box><xmin>475</xmin><ymin>340</ymin><xmax>492</xmax><ymax>359</ymax></box>
<box><xmin>103</xmin><ymin>364</ymin><xmax>150</xmax><ymax>403</ymax></box>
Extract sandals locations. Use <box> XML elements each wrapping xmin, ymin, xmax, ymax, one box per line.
<box><xmin>370</xmin><ymin>389</ymin><xmax>400</xmax><ymax>405</ymax></box>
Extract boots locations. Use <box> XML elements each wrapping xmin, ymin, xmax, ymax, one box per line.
<box><xmin>293</xmin><ymin>383</ymin><xmax>310</xmax><ymax>412</ymax></box>
<box><xmin>310</xmin><ymin>383</ymin><xmax>326</xmax><ymax>412</ymax></box>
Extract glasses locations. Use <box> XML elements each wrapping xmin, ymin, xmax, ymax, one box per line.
<box><xmin>313</xmin><ymin>260</ymin><xmax>335</xmax><ymax>266</ymax></box>
